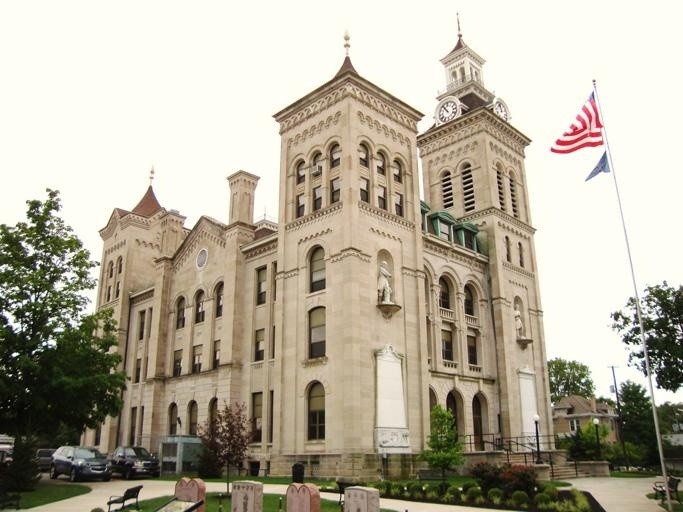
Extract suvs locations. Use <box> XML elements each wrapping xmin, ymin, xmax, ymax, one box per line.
<box><xmin>49</xmin><ymin>444</ymin><xmax>113</xmax><ymax>482</ymax></box>
<box><xmin>30</xmin><ymin>446</ymin><xmax>56</xmax><ymax>472</ymax></box>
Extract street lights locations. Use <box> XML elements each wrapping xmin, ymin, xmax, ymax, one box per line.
<box><xmin>174</xmin><ymin>416</ymin><xmax>182</xmax><ymax>474</ymax></box>
<box><xmin>592</xmin><ymin>418</ymin><xmax>603</xmax><ymax>461</ymax></box>
<box><xmin>531</xmin><ymin>413</ymin><xmax>542</xmax><ymax>463</ymax></box>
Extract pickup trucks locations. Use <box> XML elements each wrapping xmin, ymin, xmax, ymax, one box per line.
<box><xmin>107</xmin><ymin>446</ymin><xmax>162</xmax><ymax>481</ymax></box>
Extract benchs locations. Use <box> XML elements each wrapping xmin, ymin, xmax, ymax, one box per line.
<box><xmin>653</xmin><ymin>476</ymin><xmax>681</xmax><ymax>506</ymax></box>
<box><xmin>336</xmin><ymin>481</ymin><xmax>360</xmax><ymax>506</ymax></box>
<box><xmin>0</xmin><ymin>489</ymin><xmax>22</xmax><ymax>511</ymax></box>
<box><xmin>107</xmin><ymin>485</ymin><xmax>143</xmax><ymax>512</ymax></box>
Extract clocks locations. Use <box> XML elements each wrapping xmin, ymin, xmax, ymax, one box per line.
<box><xmin>493</xmin><ymin>96</ymin><xmax>508</xmax><ymax>122</ymax></box>
<box><xmin>435</xmin><ymin>96</ymin><xmax>462</xmax><ymax>127</ymax></box>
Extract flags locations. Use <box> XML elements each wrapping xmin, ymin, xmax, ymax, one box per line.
<box><xmin>549</xmin><ymin>90</ymin><xmax>608</xmax><ymax>154</ymax></box>
<box><xmin>582</xmin><ymin>148</ymin><xmax>611</xmax><ymax>180</ymax></box>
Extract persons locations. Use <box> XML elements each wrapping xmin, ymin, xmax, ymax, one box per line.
<box><xmin>513</xmin><ymin>303</ymin><xmax>527</xmax><ymax>338</ymax></box>
<box><xmin>376</xmin><ymin>257</ymin><xmax>396</xmax><ymax>304</ymax></box>
